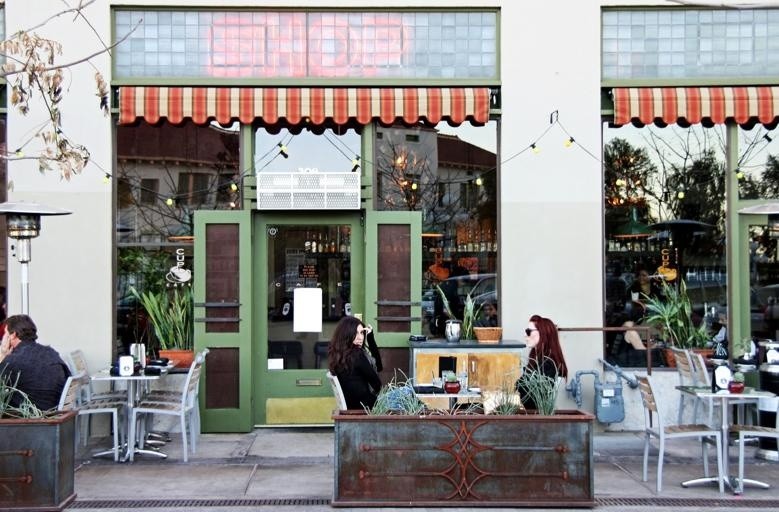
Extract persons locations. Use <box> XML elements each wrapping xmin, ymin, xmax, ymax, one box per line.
<box><xmin>0</xmin><ymin>315</ymin><xmax>71</xmax><ymax>419</ymax></box>
<box><xmin>328</xmin><ymin>316</ymin><xmax>382</xmax><ymax>411</ymax></box>
<box><xmin>605</xmin><ymin>268</ymin><xmax>760</xmax><ymax>371</ymax></box>
<box><xmin>435</xmin><ymin>265</ymin><xmax>469</xmax><ymax>339</ymax></box>
<box><xmin>479</xmin><ymin>299</ymin><xmax>497</xmax><ymax>327</ymax></box>
<box><xmin>513</xmin><ymin>316</ymin><xmax>568</xmax><ymax>411</ymax></box>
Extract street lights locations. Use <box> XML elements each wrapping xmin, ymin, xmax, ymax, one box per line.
<box><xmin>0</xmin><ymin>203</ymin><xmax>74</xmax><ymax>315</ymax></box>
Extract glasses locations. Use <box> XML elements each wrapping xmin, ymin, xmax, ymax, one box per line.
<box><xmin>525</xmin><ymin>327</ymin><xmax>538</xmax><ymax>336</ymax></box>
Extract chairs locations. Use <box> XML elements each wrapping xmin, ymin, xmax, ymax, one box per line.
<box><xmin>326</xmin><ymin>369</ymin><xmax>348</xmax><ymax>412</ymax></box>
<box><xmin>635</xmin><ymin>348</ymin><xmax>779</xmax><ymax>498</ymax></box>
<box><xmin>59</xmin><ymin>349</ymin><xmax>210</xmax><ymax>466</ymax></box>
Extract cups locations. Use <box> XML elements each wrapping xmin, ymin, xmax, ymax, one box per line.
<box><xmin>458</xmin><ymin>374</ymin><xmax>468</xmax><ymax>395</ymax></box>
<box><xmin>432</xmin><ymin>378</ymin><xmax>444</xmax><ymax>395</ymax></box>
<box><xmin>446</xmin><ymin>319</ymin><xmax>462</xmax><ymax>341</ymax></box>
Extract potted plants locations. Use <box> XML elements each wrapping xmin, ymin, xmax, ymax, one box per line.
<box><xmin>444</xmin><ymin>370</ymin><xmax>460</xmax><ymax>394</ymax></box>
<box><xmin>640</xmin><ymin>274</ymin><xmax>718</xmax><ymax>369</ymax></box>
<box><xmin>0</xmin><ymin>364</ymin><xmax>81</xmax><ymax>512</ymax></box>
<box><xmin>129</xmin><ymin>280</ymin><xmax>196</xmax><ymax>368</ymax></box>
<box><xmin>331</xmin><ymin>344</ymin><xmax>595</xmax><ymax>510</ymax></box>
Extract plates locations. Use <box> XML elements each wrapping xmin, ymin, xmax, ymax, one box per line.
<box><xmin>468</xmin><ymin>387</ymin><xmax>481</xmax><ymax>393</ymax></box>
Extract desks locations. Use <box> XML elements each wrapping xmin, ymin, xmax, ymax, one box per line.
<box><xmin>414</xmin><ymin>383</ymin><xmax>481</xmax><ymax>409</ymax></box>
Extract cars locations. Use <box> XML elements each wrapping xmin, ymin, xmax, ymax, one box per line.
<box><xmin>421</xmin><ymin>273</ymin><xmax>498</xmax><ymax>334</ymax></box>
<box><xmin>718</xmin><ymin>284</ymin><xmax>779</xmax><ymax>334</ymax></box>
<box><xmin>268</xmin><ymin>277</ymin><xmax>328</xmax><ymax>319</ymax></box>
<box><xmin>117</xmin><ymin>287</ymin><xmax>150</xmax><ymax>352</ymax></box>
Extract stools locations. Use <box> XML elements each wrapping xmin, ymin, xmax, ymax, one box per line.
<box><xmin>314</xmin><ymin>340</ymin><xmax>331</xmax><ymax>369</ymax></box>
<box><xmin>272</xmin><ymin>340</ymin><xmax>303</xmax><ymax>368</ymax></box>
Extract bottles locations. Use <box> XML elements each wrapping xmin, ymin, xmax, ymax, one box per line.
<box><xmin>436</xmin><ymin>224</ymin><xmax>500</xmax><ymax>254</ymax></box>
<box><xmin>608</xmin><ymin>229</ymin><xmax>674</xmax><ymax>252</ymax></box>
<box><xmin>304</xmin><ymin>230</ymin><xmax>351</xmax><ymax>253</ymax></box>
